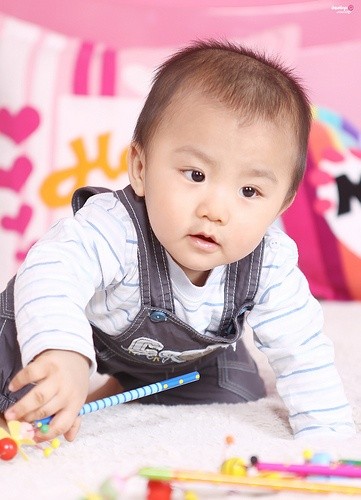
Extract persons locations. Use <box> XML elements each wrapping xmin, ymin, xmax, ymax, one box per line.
<box><xmin>0</xmin><ymin>37</ymin><xmax>361</xmax><ymax>464</ymax></box>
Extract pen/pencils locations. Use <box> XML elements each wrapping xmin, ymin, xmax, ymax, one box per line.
<box><xmin>34</xmin><ymin>370</ymin><xmax>201</xmax><ymax>430</ymax></box>
<box><xmin>138</xmin><ymin>466</ymin><xmax>361</xmax><ymax>498</ymax></box>
<box><xmin>253</xmin><ymin>462</ymin><xmax>361</xmax><ymax>480</ymax></box>
